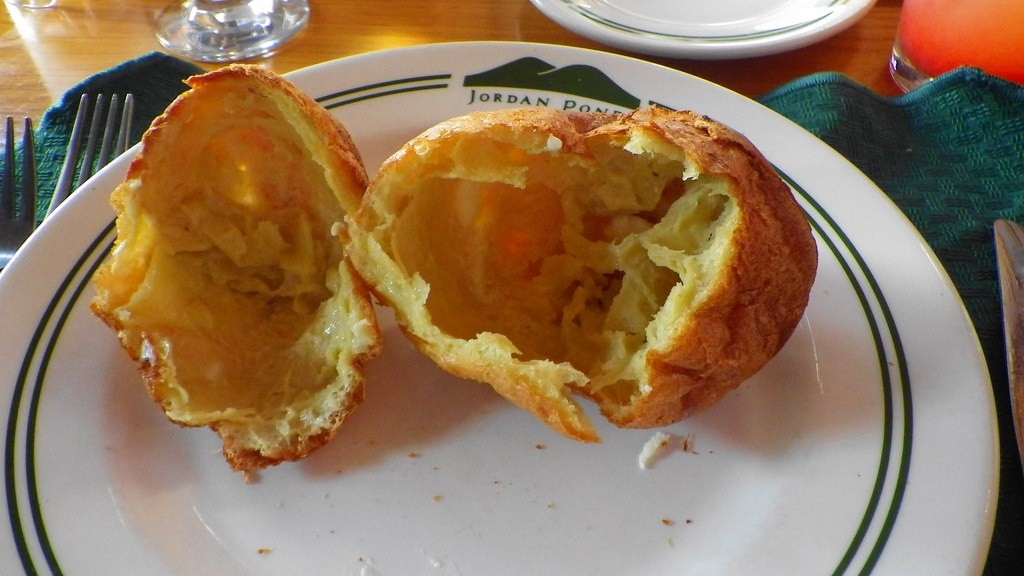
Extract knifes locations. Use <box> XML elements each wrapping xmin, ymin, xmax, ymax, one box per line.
<box><xmin>994</xmin><ymin>220</ymin><xmax>1024</xmax><ymax>460</ymax></box>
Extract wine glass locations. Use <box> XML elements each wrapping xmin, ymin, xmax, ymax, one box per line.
<box><xmin>155</xmin><ymin>0</ymin><xmax>309</xmax><ymax>62</ymax></box>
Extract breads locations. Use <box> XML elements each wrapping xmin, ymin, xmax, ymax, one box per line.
<box><xmin>85</xmin><ymin>65</ymin><xmax>818</xmax><ymax>472</ymax></box>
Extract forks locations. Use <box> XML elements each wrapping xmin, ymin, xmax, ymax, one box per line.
<box><xmin>45</xmin><ymin>93</ymin><xmax>133</xmax><ymax>219</ymax></box>
<box><xmin>0</xmin><ymin>116</ymin><xmax>34</xmax><ymax>272</ymax></box>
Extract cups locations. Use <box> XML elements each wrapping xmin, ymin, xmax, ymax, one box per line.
<box><xmin>889</xmin><ymin>0</ymin><xmax>1024</xmax><ymax>94</ymax></box>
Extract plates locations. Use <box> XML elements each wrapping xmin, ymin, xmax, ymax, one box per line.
<box><xmin>0</xmin><ymin>42</ymin><xmax>998</xmax><ymax>576</ymax></box>
<box><xmin>528</xmin><ymin>0</ymin><xmax>877</xmax><ymax>59</ymax></box>
